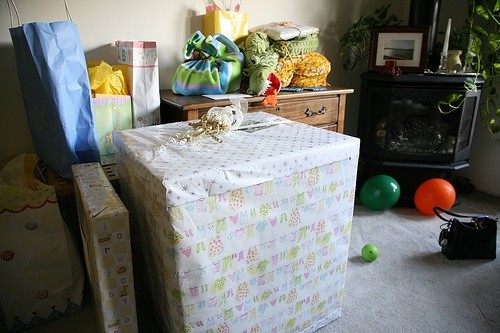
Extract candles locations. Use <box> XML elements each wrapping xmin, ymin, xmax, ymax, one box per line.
<box><xmin>443</xmin><ymin>18</ymin><xmax>451</xmax><ymax>53</ymax></box>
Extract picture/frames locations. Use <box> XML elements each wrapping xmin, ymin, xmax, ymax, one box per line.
<box><xmin>368</xmin><ymin>25</ymin><xmax>432</xmax><ymax>74</ymax></box>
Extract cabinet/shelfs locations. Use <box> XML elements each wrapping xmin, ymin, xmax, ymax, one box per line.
<box><xmin>357</xmin><ymin>74</ymin><xmax>485</xmax><ymax>208</ymax></box>
<box><xmin>160</xmin><ymin>87</ymin><xmax>355</xmax><ymax>134</ymax></box>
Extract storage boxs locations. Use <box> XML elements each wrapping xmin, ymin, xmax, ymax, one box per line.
<box><xmin>116</xmin><ymin>111</ymin><xmax>360</xmax><ymax>333</ymax></box>
<box><xmin>71</xmin><ymin>163</ymin><xmax>138</xmax><ymax>333</ymax></box>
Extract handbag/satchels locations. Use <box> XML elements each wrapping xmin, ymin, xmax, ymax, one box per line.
<box><xmin>91</xmin><ymin>90</ymin><xmax>133</xmax><ymax>165</ymax></box>
<box><xmin>6</xmin><ymin>0</ymin><xmax>101</xmax><ymax>179</ymax></box>
<box><xmin>115</xmin><ymin>41</ymin><xmax>160</xmax><ymax>128</ymax></box>
<box><xmin>203</xmin><ymin>0</ymin><xmax>248</xmax><ymax>43</ymax></box>
<box><xmin>432</xmin><ymin>206</ymin><xmax>498</xmax><ymax>260</ymax></box>
